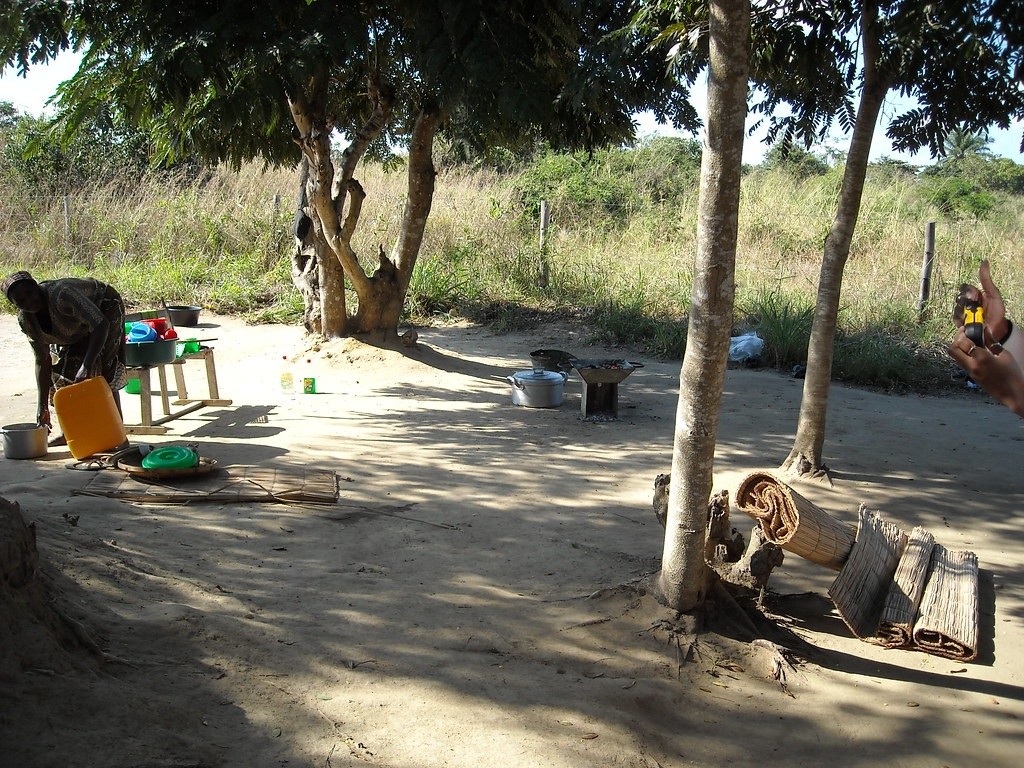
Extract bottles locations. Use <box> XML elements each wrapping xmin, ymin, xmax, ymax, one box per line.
<box><xmin>303</xmin><ymin>359</ymin><xmax>316</xmax><ymax>394</ymax></box>
<box><xmin>280</xmin><ymin>356</ymin><xmax>295</xmax><ymax>393</ymax></box>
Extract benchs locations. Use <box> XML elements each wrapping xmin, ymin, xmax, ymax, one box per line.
<box><xmin>123</xmin><ymin>309</ymin><xmax>233</xmax><ymax>434</ymax></box>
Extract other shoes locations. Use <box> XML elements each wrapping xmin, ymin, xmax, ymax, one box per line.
<box><xmin>48</xmin><ymin>434</ymin><xmax>66</xmax><ymax>447</ymax></box>
<box><xmin>107</xmin><ymin>438</ymin><xmax>130</xmax><ymax>451</ymax></box>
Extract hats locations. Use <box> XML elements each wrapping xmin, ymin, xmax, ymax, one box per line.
<box><xmin>0</xmin><ymin>269</ymin><xmax>34</xmax><ymax>302</ymax></box>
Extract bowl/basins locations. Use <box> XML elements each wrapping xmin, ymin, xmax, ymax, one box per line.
<box><xmin>123</xmin><ymin>338</ymin><xmax>179</xmax><ymax>366</ymax></box>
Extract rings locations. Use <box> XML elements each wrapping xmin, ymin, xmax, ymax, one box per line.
<box><xmin>989</xmin><ymin>342</ymin><xmax>1001</xmax><ymax>347</ymax></box>
<box><xmin>968</xmin><ymin>345</ymin><xmax>976</xmax><ymax>354</ymax></box>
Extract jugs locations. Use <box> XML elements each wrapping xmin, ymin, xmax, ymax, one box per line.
<box><xmin>185</xmin><ymin>338</ymin><xmax>201</xmax><ymax>353</ymax></box>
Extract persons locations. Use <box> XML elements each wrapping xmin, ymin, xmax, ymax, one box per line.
<box><xmin>1</xmin><ymin>271</ymin><xmax>130</xmax><ymax>453</ymax></box>
<box><xmin>948</xmin><ymin>260</ymin><xmax>1024</xmax><ymax>418</ymax></box>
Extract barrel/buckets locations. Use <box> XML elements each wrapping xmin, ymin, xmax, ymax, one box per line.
<box><xmin>55</xmin><ymin>376</ymin><xmax>126</xmax><ymax>460</ymax></box>
<box><xmin>142</xmin><ymin>319</ymin><xmax>167</xmax><ymax>335</ymax></box>
<box><xmin>124</xmin><ymin>379</ymin><xmax>141</xmax><ymax>393</ymax></box>
<box><xmin>529</xmin><ymin>350</ymin><xmax>577</xmax><ymax>386</ymax></box>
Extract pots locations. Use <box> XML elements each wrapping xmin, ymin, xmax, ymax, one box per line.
<box><xmin>507</xmin><ymin>369</ymin><xmax>569</xmax><ymax>407</ymax></box>
<box><xmin>0</xmin><ymin>423</ymin><xmax>52</xmax><ymax>459</ymax></box>
